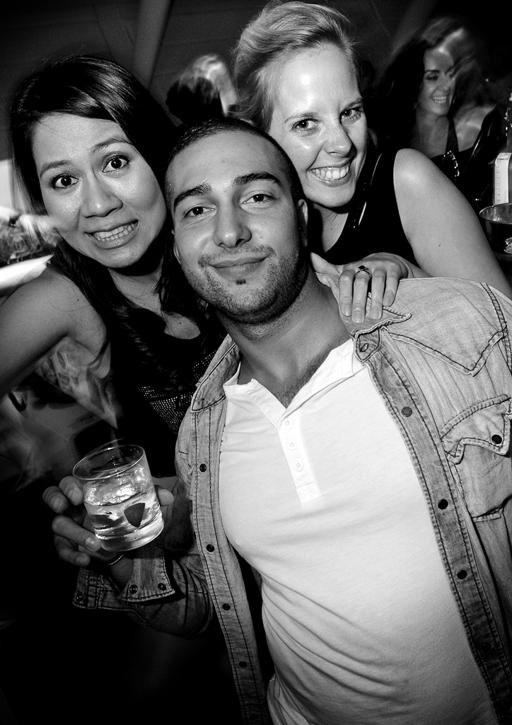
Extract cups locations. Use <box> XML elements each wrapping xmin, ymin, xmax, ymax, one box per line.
<box><xmin>69</xmin><ymin>443</ymin><xmax>166</xmax><ymax>552</ymax></box>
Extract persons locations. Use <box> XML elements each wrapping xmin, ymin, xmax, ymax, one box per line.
<box><xmin>1</xmin><ymin>3</ymin><xmax>512</xmax><ymax>725</ymax></box>
